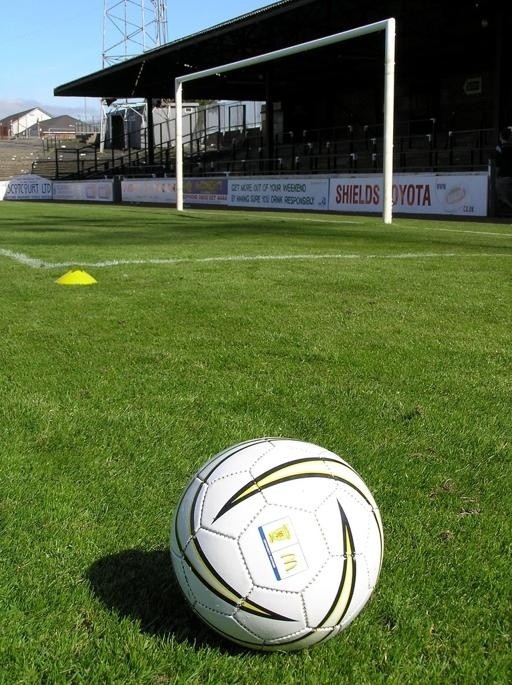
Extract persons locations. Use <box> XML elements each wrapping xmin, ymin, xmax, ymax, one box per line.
<box><xmin>232</xmin><ymin>135</ymin><xmax>236</xmax><ymax>144</ymax></box>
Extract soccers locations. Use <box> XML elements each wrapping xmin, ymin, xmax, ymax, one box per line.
<box><xmin>170</xmin><ymin>437</ymin><xmax>383</xmax><ymax>651</ymax></box>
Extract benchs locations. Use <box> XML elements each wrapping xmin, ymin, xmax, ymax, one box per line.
<box><xmin>113</xmin><ymin>125</ymin><xmax>495</xmax><ymax>174</ymax></box>
